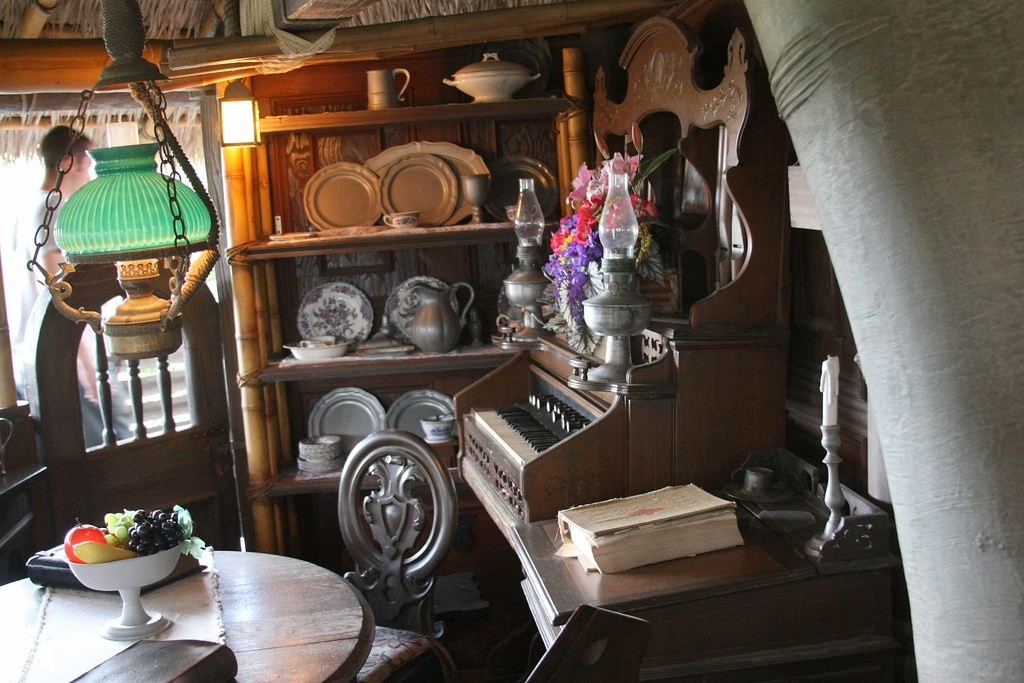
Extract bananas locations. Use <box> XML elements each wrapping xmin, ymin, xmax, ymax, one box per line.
<box><xmin>72</xmin><ymin>542</ymin><xmax>138</xmax><ymax>564</ymax></box>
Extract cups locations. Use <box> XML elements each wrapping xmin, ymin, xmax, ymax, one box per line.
<box><xmin>365</xmin><ymin>68</ymin><xmax>409</xmax><ymax>110</ymax></box>
<box><xmin>506</xmin><ymin>205</ymin><xmax>538</xmax><ymax>223</ymax></box>
<box><xmin>383</xmin><ymin>210</ymin><xmax>420</xmax><ymax>228</ymax></box>
<box><xmin>742</xmin><ymin>467</ymin><xmax>774</xmax><ymax>495</ymax></box>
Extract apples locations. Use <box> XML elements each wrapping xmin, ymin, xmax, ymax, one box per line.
<box><xmin>64</xmin><ymin>518</ymin><xmax>106</xmax><ymax>563</ymax></box>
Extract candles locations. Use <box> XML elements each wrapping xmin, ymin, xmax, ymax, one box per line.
<box><xmin>820</xmin><ymin>354</ymin><xmax>839</xmax><ymax>425</ymax></box>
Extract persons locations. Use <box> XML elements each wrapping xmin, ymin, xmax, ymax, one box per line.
<box><xmin>28</xmin><ymin>125</ymin><xmax>130</xmax><ymax>448</ymax></box>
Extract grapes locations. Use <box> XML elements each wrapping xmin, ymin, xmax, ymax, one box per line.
<box><xmin>105</xmin><ymin>510</ymin><xmax>183</xmax><ymax>556</ymax></box>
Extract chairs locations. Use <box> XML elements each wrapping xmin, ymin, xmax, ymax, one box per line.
<box><xmin>337</xmin><ymin>430</ymin><xmax>459</xmax><ymax>683</ymax></box>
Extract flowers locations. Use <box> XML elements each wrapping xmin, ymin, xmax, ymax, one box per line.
<box><xmin>543</xmin><ymin>122</ymin><xmax>678</xmax><ymax>356</ymax></box>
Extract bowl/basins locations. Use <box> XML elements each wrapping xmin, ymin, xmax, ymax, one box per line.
<box><xmin>420</xmin><ymin>414</ymin><xmax>455</xmax><ymax>443</ymax></box>
<box><xmin>282</xmin><ymin>338</ymin><xmax>355</xmax><ymax>360</ymax></box>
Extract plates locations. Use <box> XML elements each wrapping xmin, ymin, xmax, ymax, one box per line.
<box><xmin>296</xmin><ymin>282</ymin><xmax>374</xmax><ymax>352</ymax></box>
<box><xmin>297</xmin><ymin>456</ymin><xmax>342</xmax><ymax>472</ymax></box>
<box><xmin>385</xmin><ymin>276</ymin><xmax>459</xmax><ymax>345</ymax></box>
<box><xmin>299</xmin><ymin>434</ymin><xmax>342</xmax><ymax>461</ymax></box>
<box><xmin>60</xmin><ymin>538</ymin><xmax>189</xmax><ymax>641</ymax></box>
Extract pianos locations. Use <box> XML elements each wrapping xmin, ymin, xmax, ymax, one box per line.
<box><xmin>449</xmin><ymin>339</ymin><xmax>793</xmax><ymax>681</ymax></box>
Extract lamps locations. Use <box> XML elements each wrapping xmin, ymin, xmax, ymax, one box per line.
<box><xmin>217</xmin><ymin>75</ymin><xmax>262</xmax><ymax>147</ymax></box>
<box><xmin>28</xmin><ymin>75</ymin><xmax>220</xmax><ymax>358</ymax></box>
<box><xmin>568</xmin><ymin>171</ymin><xmax>658</xmax><ymax>392</ymax></box>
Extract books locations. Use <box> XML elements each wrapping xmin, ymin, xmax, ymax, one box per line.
<box><xmin>558</xmin><ymin>482</ymin><xmax>745</xmax><ymax>574</ymax></box>
<box><xmin>26</xmin><ymin>544</ymin><xmax>208</xmax><ymax>596</ymax></box>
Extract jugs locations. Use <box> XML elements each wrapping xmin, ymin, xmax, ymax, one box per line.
<box><xmin>404</xmin><ymin>281</ymin><xmax>475</xmax><ymax>355</ymax></box>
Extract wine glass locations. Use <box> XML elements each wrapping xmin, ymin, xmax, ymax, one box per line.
<box><xmin>458</xmin><ymin>173</ymin><xmax>492</xmax><ymax>224</ymax></box>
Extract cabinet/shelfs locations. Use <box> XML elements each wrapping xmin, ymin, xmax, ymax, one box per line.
<box><xmin>223</xmin><ymin>46</ymin><xmax>586</xmax><ymax>557</ymax></box>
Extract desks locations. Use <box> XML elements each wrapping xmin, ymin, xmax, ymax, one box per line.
<box><xmin>0</xmin><ymin>550</ymin><xmax>376</xmax><ymax>683</ymax></box>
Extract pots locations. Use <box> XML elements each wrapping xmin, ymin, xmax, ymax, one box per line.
<box><xmin>443</xmin><ymin>53</ymin><xmax>541</xmax><ymax>103</ymax></box>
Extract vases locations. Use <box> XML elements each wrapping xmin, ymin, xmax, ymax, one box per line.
<box><xmin>396</xmin><ymin>281</ymin><xmax>475</xmax><ymax>355</ymax></box>
